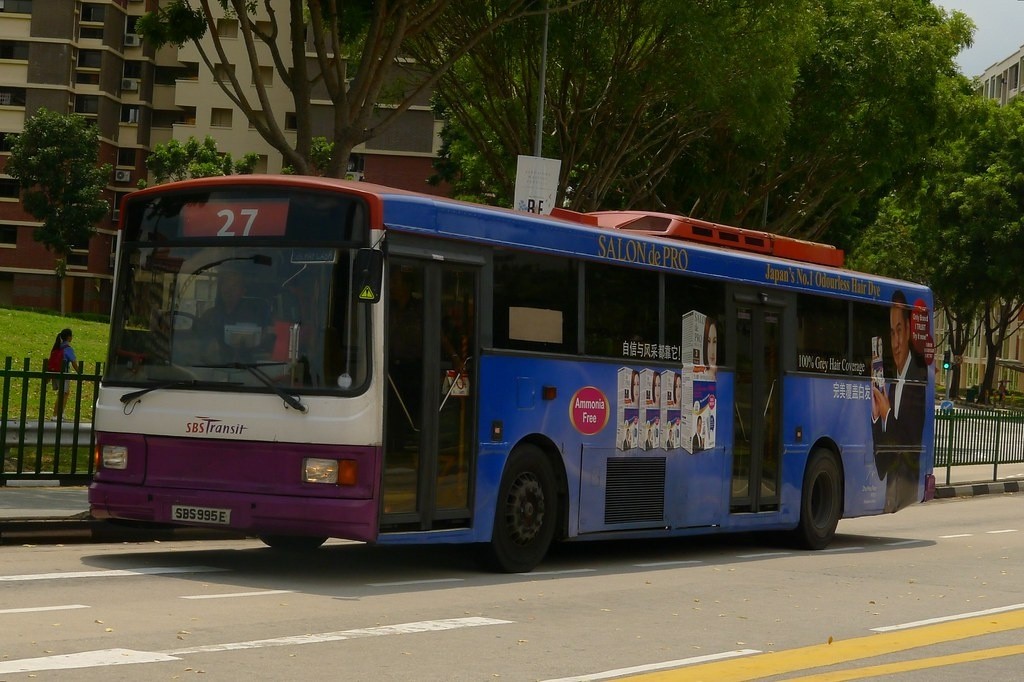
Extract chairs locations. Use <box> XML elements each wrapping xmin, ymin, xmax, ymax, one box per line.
<box><xmin>236</xmin><ymin>296</ymin><xmax>275</xmax><ymax>351</ymax></box>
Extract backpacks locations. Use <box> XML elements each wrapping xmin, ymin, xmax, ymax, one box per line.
<box><xmin>48</xmin><ymin>345</ymin><xmax>70</xmax><ymax>371</ymax></box>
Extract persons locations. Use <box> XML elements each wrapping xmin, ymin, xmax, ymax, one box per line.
<box><xmin>668</xmin><ymin>373</ymin><xmax>682</xmax><ymax>409</ymax></box>
<box><xmin>196</xmin><ymin>266</ymin><xmax>870</xmax><ymax>504</ymax></box>
<box><xmin>667</xmin><ymin>430</ymin><xmax>674</xmax><ymax>448</ymax></box>
<box><xmin>694</xmin><ymin>316</ymin><xmax>717</xmax><ymax>381</ymax></box>
<box><xmin>692</xmin><ymin>415</ymin><xmax>704</xmax><ymax>454</ymax></box>
<box><xmin>625</xmin><ymin>370</ymin><xmax>640</xmax><ymax>408</ymax></box>
<box><xmin>47</xmin><ymin>328</ymin><xmax>84</xmax><ymax>422</ymax></box>
<box><xmin>646</xmin><ymin>429</ymin><xmax>653</xmax><ymax>448</ymax></box>
<box><xmin>646</xmin><ymin>371</ymin><xmax>661</xmax><ymax>408</ymax></box>
<box><xmin>623</xmin><ymin>429</ymin><xmax>631</xmax><ymax>451</ymax></box>
<box><xmin>870</xmin><ymin>290</ymin><xmax>926</xmax><ymax>514</ymax></box>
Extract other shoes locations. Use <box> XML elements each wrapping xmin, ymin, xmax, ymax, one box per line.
<box><xmin>53</xmin><ymin>417</ymin><xmax>63</xmax><ymax>421</ymax></box>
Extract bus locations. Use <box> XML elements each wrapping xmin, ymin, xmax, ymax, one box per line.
<box><xmin>85</xmin><ymin>174</ymin><xmax>937</xmax><ymax>573</ymax></box>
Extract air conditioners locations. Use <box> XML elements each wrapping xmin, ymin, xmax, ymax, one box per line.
<box><xmin>123</xmin><ymin>34</ymin><xmax>141</xmax><ymax>47</ymax></box>
<box><xmin>121</xmin><ymin>78</ymin><xmax>137</xmax><ymax>91</ymax></box>
<box><xmin>345</xmin><ymin>172</ymin><xmax>361</xmax><ymax>181</ymax></box>
<box><xmin>115</xmin><ymin>170</ymin><xmax>131</xmax><ymax>182</ymax></box>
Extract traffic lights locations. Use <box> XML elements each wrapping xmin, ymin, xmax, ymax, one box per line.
<box><xmin>943</xmin><ymin>351</ymin><xmax>951</xmax><ymax>370</ymax></box>
